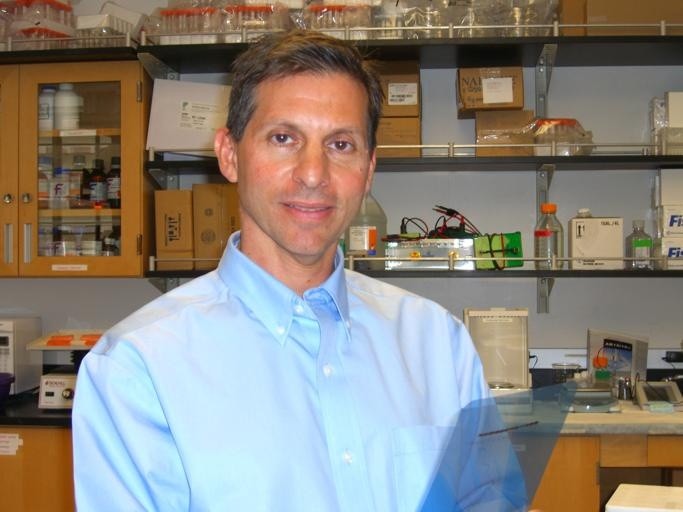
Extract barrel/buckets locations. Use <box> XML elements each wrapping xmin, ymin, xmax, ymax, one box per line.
<box><xmin>345</xmin><ymin>193</ymin><xmax>388</xmax><ymax>270</ymax></box>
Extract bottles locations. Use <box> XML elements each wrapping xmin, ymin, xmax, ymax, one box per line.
<box><xmin>532</xmin><ymin>200</ymin><xmax>564</xmax><ymax>269</ymax></box>
<box><xmin>625</xmin><ymin>217</ymin><xmax>654</xmax><ymax>267</ymax></box>
<box><xmin>36</xmin><ymin>82</ymin><xmax>122</xmax><ymax>257</ymax></box>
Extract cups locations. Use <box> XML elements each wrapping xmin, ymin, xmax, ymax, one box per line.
<box><xmin>552</xmin><ymin>362</ymin><xmax>581</xmax><ymax>385</ymax></box>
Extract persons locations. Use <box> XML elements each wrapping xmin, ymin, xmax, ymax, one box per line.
<box><xmin>70</xmin><ymin>29</ymin><xmax>527</xmax><ymax>511</ymax></box>
